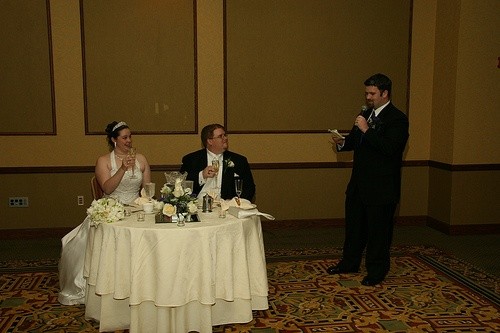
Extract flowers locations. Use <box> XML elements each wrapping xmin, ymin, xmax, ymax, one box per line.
<box><xmin>225</xmin><ymin>156</ymin><xmax>235</xmax><ymax>168</ymax></box>
<box><xmin>153</xmin><ymin>178</ymin><xmax>199</xmax><ymax>217</ymax></box>
<box><xmin>86</xmin><ymin>195</ymin><xmax>125</xmax><ymax>229</ymax></box>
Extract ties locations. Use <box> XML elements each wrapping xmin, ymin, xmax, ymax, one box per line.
<box><xmin>367</xmin><ymin>111</ymin><xmax>375</xmax><ymax>126</ymax></box>
<box><xmin>210</xmin><ymin>156</ymin><xmax>219</xmax><ymax>191</ymax></box>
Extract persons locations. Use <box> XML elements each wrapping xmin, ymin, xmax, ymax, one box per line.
<box><xmin>180</xmin><ymin>124</ymin><xmax>256</xmax><ymax>203</ymax></box>
<box><xmin>57</xmin><ymin>121</ymin><xmax>152</xmax><ymax>305</ymax></box>
<box><xmin>328</xmin><ymin>74</ymin><xmax>409</xmax><ymax>284</ymax></box>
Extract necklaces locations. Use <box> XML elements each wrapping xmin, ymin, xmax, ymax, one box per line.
<box><xmin>114</xmin><ymin>154</ymin><xmax>126</xmax><ymax>160</ymax></box>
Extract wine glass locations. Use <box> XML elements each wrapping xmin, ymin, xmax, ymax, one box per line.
<box><xmin>235</xmin><ymin>179</ymin><xmax>243</xmax><ymax>199</ymax></box>
<box><xmin>144</xmin><ymin>183</ymin><xmax>155</xmax><ymax>203</ymax></box>
<box><xmin>212</xmin><ymin>161</ymin><xmax>220</xmax><ymax>189</ymax></box>
<box><xmin>127</xmin><ymin>148</ymin><xmax>137</xmax><ymax>179</ymax></box>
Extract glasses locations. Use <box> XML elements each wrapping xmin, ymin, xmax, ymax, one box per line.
<box><xmin>209</xmin><ymin>133</ymin><xmax>228</xmax><ymax>140</ymax></box>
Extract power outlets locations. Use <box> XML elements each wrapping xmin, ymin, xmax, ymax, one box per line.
<box><xmin>78</xmin><ymin>196</ymin><xmax>84</xmax><ymax>205</ymax></box>
<box><xmin>9</xmin><ymin>197</ymin><xmax>28</xmax><ymax>208</ymax></box>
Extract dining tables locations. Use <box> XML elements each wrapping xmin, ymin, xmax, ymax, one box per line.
<box><xmin>83</xmin><ymin>202</ymin><xmax>269</xmax><ymax>333</ymax></box>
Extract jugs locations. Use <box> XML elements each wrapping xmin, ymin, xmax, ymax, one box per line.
<box><xmin>202</xmin><ymin>193</ymin><xmax>213</xmax><ymax>213</ymax></box>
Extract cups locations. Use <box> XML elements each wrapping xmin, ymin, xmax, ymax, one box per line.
<box><xmin>137</xmin><ymin>213</ymin><xmax>145</xmax><ymax>222</ymax></box>
<box><xmin>124</xmin><ymin>208</ymin><xmax>131</xmax><ymax>216</ymax></box>
<box><xmin>219</xmin><ymin>210</ymin><xmax>226</xmax><ymax>218</ymax></box>
<box><xmin>144</xmin><ymin>204</ymin><xmax>153</xmax><ymax>213</ymax></box>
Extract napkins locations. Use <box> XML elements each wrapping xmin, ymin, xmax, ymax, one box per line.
<box><xmin>220</xmin><ymin>197</ymin><xmax>253</xmax><ymax>211</ymax></box>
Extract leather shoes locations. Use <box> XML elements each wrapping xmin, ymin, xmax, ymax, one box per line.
<box><xmin>327</xmin><ymin>264</ymin><xmax>354</xmax><ymax>273</ymax></box>
<box><xmin>362</xmin><ymin>274</ymin><xmax>382</xmax><ymax>284</ymax></box>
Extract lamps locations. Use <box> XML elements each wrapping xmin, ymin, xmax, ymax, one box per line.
<box><xmin>134</xmin><ymin>188</ymin><xmax>149</xmax><ymax>205</ymax></box>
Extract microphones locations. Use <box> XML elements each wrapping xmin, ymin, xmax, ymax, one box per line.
<box><xmin>362</xmin><ymin>105</ymin><xmax>368</xmax><ymax>117</ymax></box>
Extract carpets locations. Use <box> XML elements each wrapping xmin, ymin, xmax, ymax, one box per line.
<box><xmin>0</xmin><ymin>243</ymin><xmax>500</xmax><ymax>333</ymax></box>
